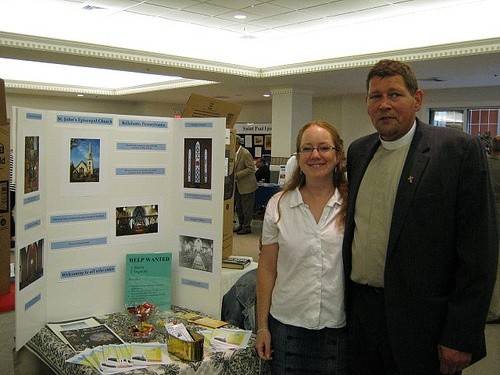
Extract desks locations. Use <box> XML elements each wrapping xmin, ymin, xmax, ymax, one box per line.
<box><xmin>233</xmin><ymin>184</ymin><xmax>280</xmax><ymax>223</ymax></box>
<box><xmin>13</xmin><ymin>304</ymin><xmax>271</xmax><ymax>375</ymax></box>
<box><xmin>221</xmin><ymin>255</ymin><xmax>259</xmax><ymax>299</ymax></box>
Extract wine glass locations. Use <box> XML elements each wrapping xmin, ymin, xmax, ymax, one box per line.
<box><xmin>126</xmin><ymin>302</ymin><xmax>156</xmax><ymax>338</ymax></box>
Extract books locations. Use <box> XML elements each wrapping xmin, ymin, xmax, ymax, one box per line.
<box><xmin>46</xmin><ymin>316</ymin><xmax>172</xmax><ymax>375</ymax></box>
<box><xmin>222</xmin><ymin>255</ymin><xmax>253</xmax><ymax>270</ymax></box>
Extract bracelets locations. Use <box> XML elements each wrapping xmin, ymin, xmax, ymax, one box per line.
<box><xmin>256</xmin><ymin>328</ymin><xmax>269</xmax><ymax>333</ymax></box>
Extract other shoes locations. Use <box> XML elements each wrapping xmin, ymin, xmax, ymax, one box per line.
<box><xmin>237</xmin><ymin>227</ymin><xmax>251</xmax><ymax>234</ymax></box>
<box><xmin>233</xmin><ymin>226</ymin><xmax>242</xmax><ymax>232</ymax></box>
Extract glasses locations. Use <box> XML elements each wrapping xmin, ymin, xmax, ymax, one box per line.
<box><xmin>298</xmin><ymin>142</ymin><xmax>339</xmax><ymax>153</ymax></box>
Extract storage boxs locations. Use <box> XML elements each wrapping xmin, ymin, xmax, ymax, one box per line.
<box><xmin>180</xmin><ymin>94</ymin><xmax>244</xmax><ymax>262</ymax></box>
<box><xmin>0</xmin><ymin>77</ymin><xmax>11</xmax><ymax>297</ymax></box>
<box><xmin>165</xmin><ymin>329</ymin><xmax>205</xmax><ymax>360</ymax></box>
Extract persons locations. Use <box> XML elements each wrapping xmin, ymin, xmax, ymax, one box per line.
<box><xmin>256</xmin><ymin>121</ymin><xmax>350</xmax><ymax>375</ymax></box>
<box><xmin>232</xmin><ymin>135</ymin><xmax>271</xmax><ymax>236</ymax></box>
<box><xmin>347</xmin><ymin>59</ymin><xmax>500</xmax><ymax>375</ymax></box>
